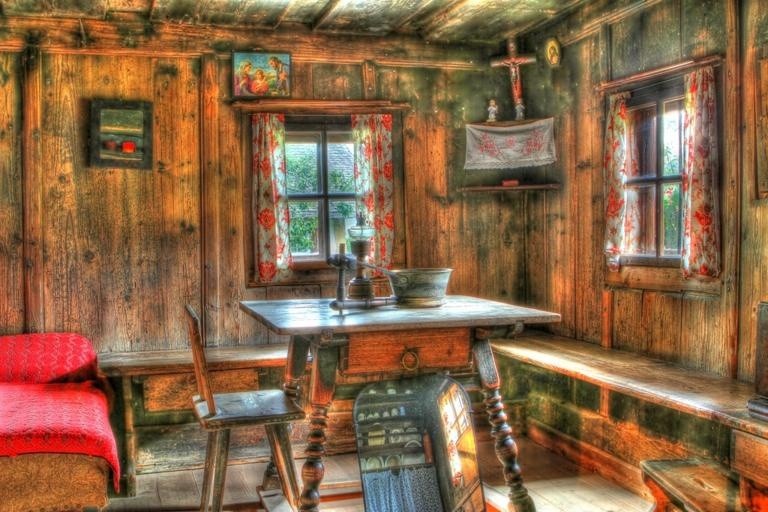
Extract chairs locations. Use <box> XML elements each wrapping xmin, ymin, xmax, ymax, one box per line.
<box><xmin>177</xmin><ymin>302</ymin><xmax>300</xmax><ymax>510</ymax></box>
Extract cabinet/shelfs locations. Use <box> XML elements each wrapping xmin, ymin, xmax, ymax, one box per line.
<box><xmin>485</xmin><ymin>351</ymin><xmax>768</xmax><ymax>506</ymax></box>
<box><xmin>132</xmin><ymin>367</ymin><xmax>273</xmax><ymax>475</ymax></box>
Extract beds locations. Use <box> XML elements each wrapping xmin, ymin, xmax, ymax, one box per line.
<box><xmin>0</xmin><ymin>330</ymin><xmax>111</xmax><ymax>511</ymax></box>
<box><xmin>241</xmin><ymin>292</ymin><xmax>563</xmax><ymax>512</ymax></box>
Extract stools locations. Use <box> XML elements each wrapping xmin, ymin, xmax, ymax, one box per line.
<box><xmin>639</xmin><ymin>456</ymin><xmax>754</xmax><ymax>512</ymax></box>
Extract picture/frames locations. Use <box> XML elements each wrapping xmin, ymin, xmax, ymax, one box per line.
<box><xmin>230</xmin><ymin>48</ymin><xmax>293</xmax><ymax>100</ymax></box>
<box><xmin>83</xmin><ymin>94</ymin><xmax>153</xmax><ymax>169</ymax></box>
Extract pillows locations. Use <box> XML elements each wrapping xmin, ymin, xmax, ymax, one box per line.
<box><xmin>0</xmin><ymin>333</ymin><xmax>96</xmax><ymax>383</ymax></box>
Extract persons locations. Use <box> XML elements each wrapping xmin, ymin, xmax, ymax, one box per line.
<box><xmin>267</xmin><ymin>56</ymin><xmax>290</xmax><ymax>96</ymax></box>
<box><xmin>503</xmin><ymin>60</ymin><xmax>521</xmax><ymax>92</ymax></box>
<box><xmin>234</xmin><ymin>58</ymin><xmax>254</xmax><ymax>97</ymax></box>
<box><xmin>486</xmin><ymin>99</ymin><xmax>500</xmax><ymax>121</ymax></box>
<box><xmin>248</xmin><ymin>69</ymin><xmax>269</xmax><ymax>97</ymax></box>
<box><xmin>513</xmin><ymin>98</ymin><xmax>527</xmax><ymax>120</ymax></box>
<box><xmin>547</xmin><ymin>45</ymin><xmax>559</xmax><ymax>65</ymax></box>
